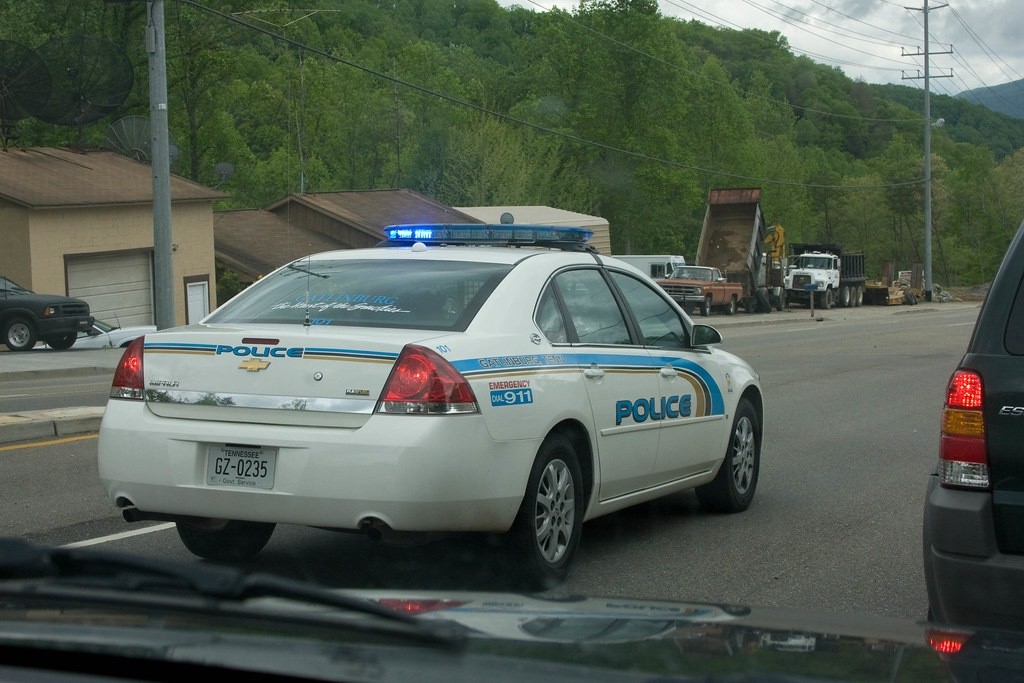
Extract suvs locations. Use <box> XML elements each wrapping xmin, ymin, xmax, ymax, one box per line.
<box><xmin>918</xmin><ymin>213</ymin><xmax>1024</xmax><ymax>683</ymax></box>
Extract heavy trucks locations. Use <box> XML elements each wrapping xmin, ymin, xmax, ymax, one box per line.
<box><xmin>784</xmin><ymin>247</ymin><xmax>867</xmax><ymax>309</ymax></box>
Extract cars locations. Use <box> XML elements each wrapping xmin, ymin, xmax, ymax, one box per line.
<box><xmin>90</xmin><ymin>221</ymin><xmax>767</xmax><ymax>596</ymax></box>
<box><xmin>0</xmin><ymin>276</ymin><xmax>94</xmax><ymax>353</ymax></box>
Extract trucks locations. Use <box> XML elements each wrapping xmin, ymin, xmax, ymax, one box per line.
<box><xmin>693</xmin><ymin>186</ymin><xmax>787</xmax><ymax>312</ymax></box>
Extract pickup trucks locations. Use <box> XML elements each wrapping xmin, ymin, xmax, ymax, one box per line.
<box><xmin>656</xmin><ymin>265</ymin><xmax>745</xmax><ymax>316</ymax></box>
